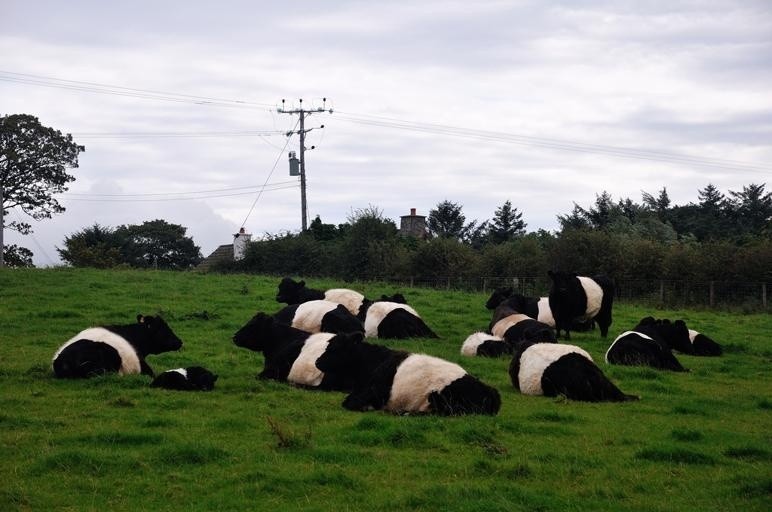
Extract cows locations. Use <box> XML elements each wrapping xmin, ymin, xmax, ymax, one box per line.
<box><xmin>51</xmin><ymin>313</ymin><xmax>184</xmax><ymax>381</ymax></box>
<box><xmin>314</xmin><ymin>330</ymin><xmax>501</xmax><ymax>417</ymax></box>
<box><xmin>149</xmin><ymin>366</ymin><xmax>219</xmax><ymax>392</ymax></box>
<box><xmin>508</xmin><ymin>327</ymin><xmax>640</xmax><ymax>403</ymax></box>
<box><xmin>232</xmin><ymin>277</ymin><xmax>439</xmax><ymax>392</ymax></box>
<box><xmin>460</xmin><ymin>275</ymin><xmax>616</xmax><ymax>361</ymax></box>
<box><xmin>604</xmin><ymin>316</ymin><xmax>723</xmax><ymax>372</ymax></box>
